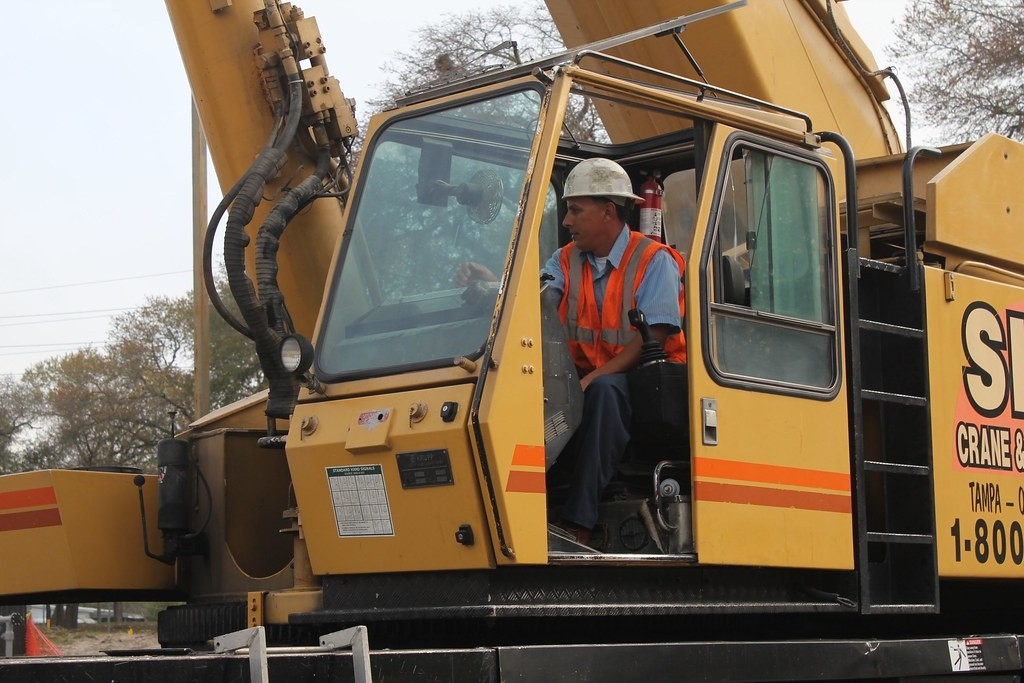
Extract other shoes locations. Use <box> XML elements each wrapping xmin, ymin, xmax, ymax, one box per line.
<box><xmin>548</xmin><ymin>523</ymin><xmax>591</xmax><ymax>551</ymax></box>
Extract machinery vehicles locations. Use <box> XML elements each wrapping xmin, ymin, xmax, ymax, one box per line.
<box><xmin>0</xmin><ymin>2</ymin><xmax>1024</xmax><ymax>683</ymax></box>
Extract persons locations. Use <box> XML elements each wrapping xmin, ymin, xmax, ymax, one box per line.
<box><xmin>456</xmin><ymin>158</ymin><xmax>685</xmax><ymax>544</ymax></box>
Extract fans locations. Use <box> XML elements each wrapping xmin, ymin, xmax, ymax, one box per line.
<box><xmin>433</xmin><ymin>166</ymin><xmax>505</xmax><ymax>224</ymax></box>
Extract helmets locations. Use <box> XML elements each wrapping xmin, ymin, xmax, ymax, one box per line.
<box><xmin>561</xmin><ymin>157</ymin><xmax>646</xmax><ymax>206</ymax></box>
